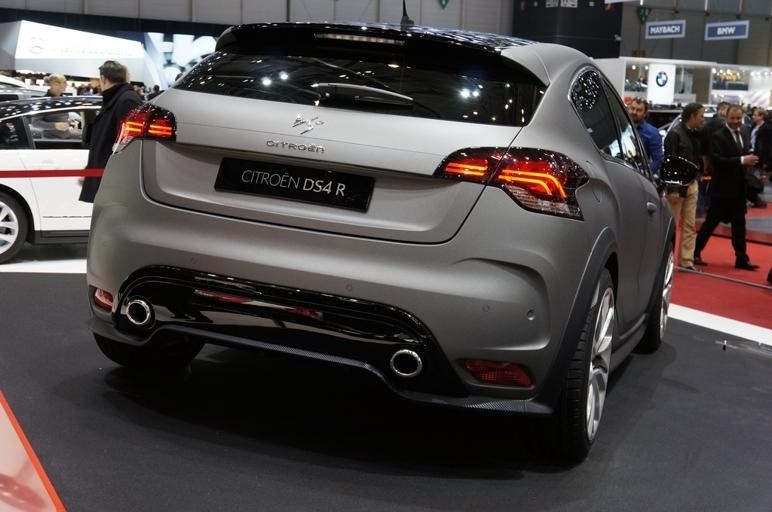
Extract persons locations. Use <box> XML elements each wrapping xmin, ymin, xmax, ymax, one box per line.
<box><xmin>29</xmin><ymin>76</ymin><xmax>39</xmax><ymax>86</ymax></box>
<box><xmin>662</xmin><ymin>103</ymin><xmax>713</xmax><ymax>274</ymax></box>
<box><xmin>71</xmin><ymin>77</ymin><xmax>164</xmax><ymax>102</ymax></box>
<box><xmin>77</xmin><ymin>59</ymin><xmax>144</xmax><ymax>204</ymax></box>
<box><xmin>690</xmin><ymin>104</ymin><xmax>762</xmax><ymax>269</ymax></box>
<box><xmin>30</xmin><ymin>72</ymin><xmax>80</xmax><ymax>140</ymax></box>
<box><xmin>41</xmin><ymin>75</ymin><xmax>51</xmax><ymax>86</ymax></box>
<box><xmin>629</xmin><ymin>96</ymin><xmax>664</xmax><ymax>176</ymax></box>
<box><xmin>20</xmin><ymin>75</ymin><xmax>25</xmax><ymax>82</ymax></box>
<box><xmin>703</xmin><ymin>102</ymin><xmax>772</xmax><ymax>208</ymax></box>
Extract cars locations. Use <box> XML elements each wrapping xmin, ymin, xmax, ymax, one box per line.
<box><xmin>85</xmin><ymin>22</ymin><xmax>679</xmax><ymax>458</ymax></box>
<box><xmin>1</xmin><ymin>75</ymin><xmax>103</xmax><ymax>263</ymax></box>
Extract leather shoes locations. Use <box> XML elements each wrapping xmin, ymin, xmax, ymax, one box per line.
<box><xmin>695</xmin><ymin>256</ymin><xmax>707</xmax><ymax>265</ymax></box>
<box><xmin>735</xmin><ymin>261</ymin><xmax>760</xmax><ymax>270</ymax></box>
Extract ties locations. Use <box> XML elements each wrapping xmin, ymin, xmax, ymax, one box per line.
<box><xmin>734</xmin><ymin>130</ymin><xmax>742</xmax><ymax>151</ymax></box>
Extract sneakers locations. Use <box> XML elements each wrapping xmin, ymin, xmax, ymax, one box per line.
<box><xmin>678</xmin><ymin>265</ymin><xmax>697</xmax><ymax>273</ymax></box>
